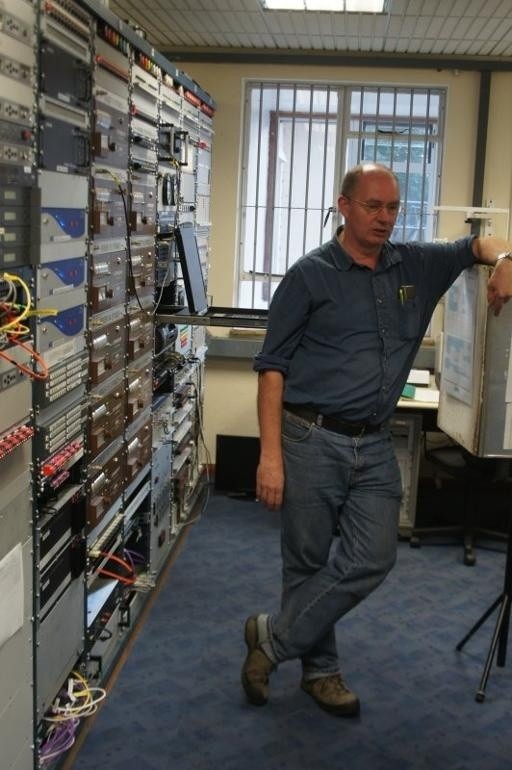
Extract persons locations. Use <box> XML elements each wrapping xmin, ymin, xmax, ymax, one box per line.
<box><xmin>241</xmin><ymin>161</ymin><xmax>512</xmax><ymax>716</ymax></box>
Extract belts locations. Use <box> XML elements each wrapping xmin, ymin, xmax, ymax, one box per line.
<box><xmin>283</xmin><ymin>403</ymin><xmax>381</xmax><ymax>436</ymax></box>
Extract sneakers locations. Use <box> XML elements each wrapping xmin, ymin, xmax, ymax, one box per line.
<box><xmin>242</xmin><ymin>616</ymin><xmax>273</xmax><ymax>700</ymax></box>
<box><xmin>300</xmin><ymin>674</ymin><xmax>360</xmax><ymax>716</ymax></box>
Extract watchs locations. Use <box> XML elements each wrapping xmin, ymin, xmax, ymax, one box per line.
<box><xmin>497</xmin><ymin>252</ymin><xmax>510</xmax><ymax>260</ymax></box>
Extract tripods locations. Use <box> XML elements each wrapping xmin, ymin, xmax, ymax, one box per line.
<box><xmin>456</xmin><ymin>522</ymin><xmax>512</xmax><ymax>702</ymax></box>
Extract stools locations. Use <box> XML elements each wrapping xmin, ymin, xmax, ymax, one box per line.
<box><xmin>409</xmin><ymin>447</ymin><xmax>509</xmax><ymax>566</ymax></box>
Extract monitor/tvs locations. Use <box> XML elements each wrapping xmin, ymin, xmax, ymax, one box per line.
<box><xmin>174</xmin><ymin>227</ymin><xmax>208</xmax><ymax>314</ymax></box>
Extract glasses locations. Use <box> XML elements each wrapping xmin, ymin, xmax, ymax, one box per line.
<box><xmin>349</xmin><ymin>197</ymin><xmax>403</xmax><ymax>215</ymax></box>
